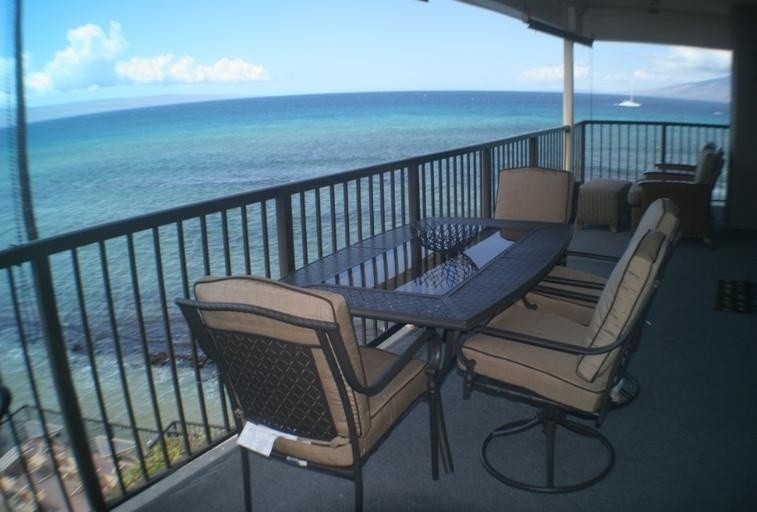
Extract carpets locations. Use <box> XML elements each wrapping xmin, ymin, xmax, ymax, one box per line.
<box><xmin>712</xmin><ymin>278</ymin><xmax>757</xmax><ymax>315</ymax></box>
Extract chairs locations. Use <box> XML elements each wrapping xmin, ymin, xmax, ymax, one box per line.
<box><xmin>492</xmin><ymin>168</ymin><xmax>575</xmax><ymax>270</ymax></box>
<box><xmin>174</xmin><ymin>273</ymin><xmax>454</xmax><ymax>510</ymax></box>
<box><xmin>499</xmin><ymin>227</ymin><xmax>526</xmax><ymax>240</ymax></box>
<box><xmin>627</xmin><ymin>141</ymin><xmax>726</xmax><ymax>238</ymax></box>
<box><xmin>516</xmin><ymin>196</ymin><xmax>678</xmax><ymax>411</ymax></box>
<box><xmin>455</xmin><ymin>229</ymin><xmax>673</xmax><ymax>495</ymax></box>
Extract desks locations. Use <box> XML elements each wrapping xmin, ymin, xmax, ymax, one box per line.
<box><xmin>275</xmin><ymin>216</ymin><xmax>576</xmax><ymax>480</ymax></box>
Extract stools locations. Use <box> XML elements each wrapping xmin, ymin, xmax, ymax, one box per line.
<box><xmin>578</xmin><ymin>176</ymin><xmax>632</xmax><ymax>231</ymax></box>
